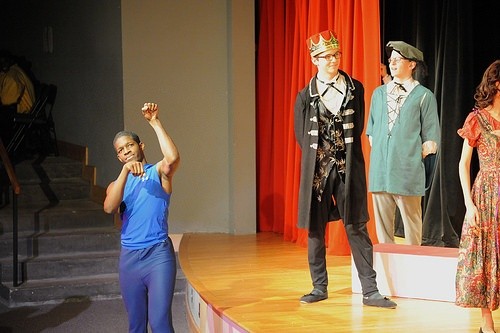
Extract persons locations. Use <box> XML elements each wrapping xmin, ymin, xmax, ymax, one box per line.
<box><xmin>365</xmin><ymin>40</ymin><xmax>441</xmax><ymax>245</ymax></box>
<box><xmin>293</xmin><ymin>30</ymin><xmax>397</xmax><ymax>309</ymax></box>
<box><xmin>380</xmin><ymin>62</ymin><xmax>392</xmax><ymax>84</ymax></box>
<box><xmin>104</xmin><ymin>102</ymin><xmax>181</xmax><ymax>333</ymax></box>
<box><xmin>0</xmin><ymin>48</ymin><xmax>36</xmax><ymax>160</ymax></box>
<box><xmin>455</xmin><ymin>59</ymin><xmax>500</xmax><ymax>333</ymax></box>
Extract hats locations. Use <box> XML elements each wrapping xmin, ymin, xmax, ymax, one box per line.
<box><xmin>386</xmin><ymin>41</ymin><xmax>423</xmax><ymax>61</ymax></box>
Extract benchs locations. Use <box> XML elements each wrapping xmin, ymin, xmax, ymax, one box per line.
<box><xmin>351</xmin><ymin>243</ymin><xmax>461</xmax><ymax>303</ymax></box>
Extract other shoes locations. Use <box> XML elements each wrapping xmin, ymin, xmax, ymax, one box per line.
<box><xmin>363</xmin><ymin>297</ymin><xmax>397</xmax><ymax>309</ymax></box>
<box><xmin>300</xmin><ymin>290</ymin><xmax>328</xmax><ymax>304</ymax></box>
<box><xmin>479</xmin><ymin>327</ymin><xmax>496</xmax><ymax>333</ymax></box>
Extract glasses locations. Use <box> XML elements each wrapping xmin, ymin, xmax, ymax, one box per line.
<box><xmin>388</xmin><ymin>57</ymin><xmax>404</xmax><ymax>63</ymax></box>
<box><xmin>315</xmin><ymin>51</ymin><xmax>342</xmax><ymax>61</ymax></box>
